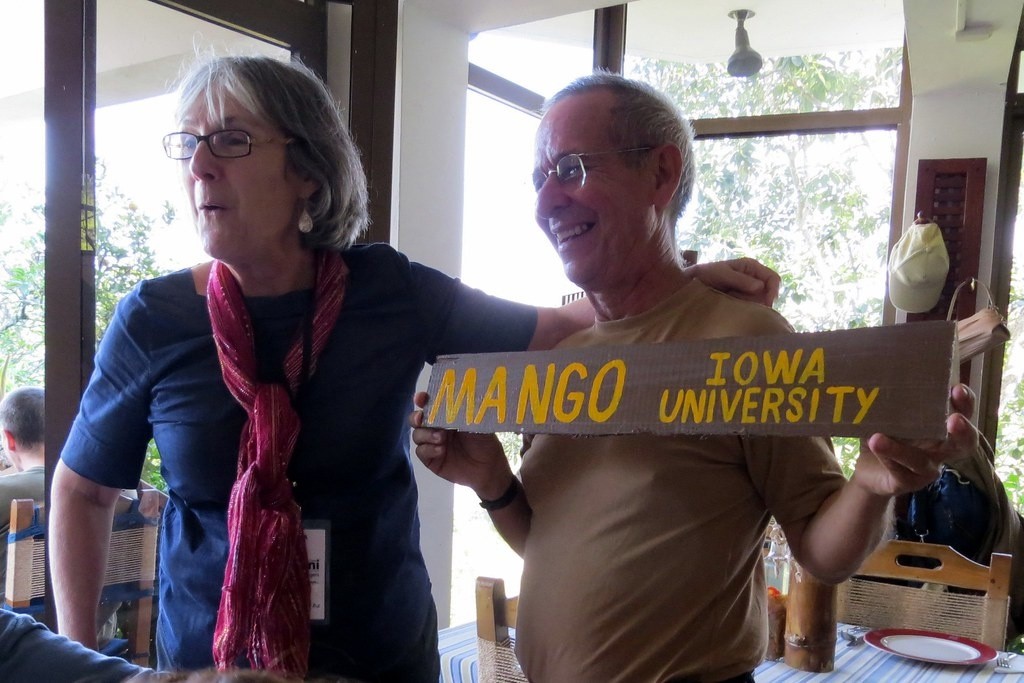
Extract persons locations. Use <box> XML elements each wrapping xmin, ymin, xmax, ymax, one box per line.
<box><xmin>0</xmin><ymin>609</ymin><xmax>282</xmax><ymax>683</ymax></box>
<box><xmin>411</xmin><ymin>72</ymin><xmax>980</xmax><ymax>683</ymax></box>
<box><xmin>0</xmin><ymin>386</ymin><xmax>139</xmax><ymax>604</ymax></box>
<box><xmin>48</xmin><ymin>32</ymin><xmax>780</xmax><ymax>683</ymax></box>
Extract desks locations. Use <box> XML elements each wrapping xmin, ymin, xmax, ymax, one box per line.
<box><xmin>439</xmin><ymin>619</ymin><xmax>1024</xmax><ymax>683</ymax></box>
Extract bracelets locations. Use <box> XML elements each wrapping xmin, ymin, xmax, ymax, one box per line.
<box><xmin>481</xmin><ymin>474</ymin><xmax>519</xmax><ymax>510</ymax></box>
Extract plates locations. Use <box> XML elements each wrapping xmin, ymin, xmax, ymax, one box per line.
<box><xmin>863</xmin><ymin>628</ymin><xmax>998</xmax><ymax>665</ymax></box>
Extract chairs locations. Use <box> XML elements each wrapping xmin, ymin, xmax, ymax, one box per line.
<box><xmin>832</xmin><ymin>538</ymin><xmax>1013</xmax><ymax>654</ymax></box>
<box><xmin>7</xmin><ymin>489</ymin><xmax>158</xmax><ymax>671</ymax></box>
<box><xmin>475</xmin><ymin>574</ymin><xmax>519</xmax><ymax>683</ymax></box>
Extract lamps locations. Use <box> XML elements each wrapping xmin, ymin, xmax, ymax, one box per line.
<box><xmin>727</xmin><ymin>8</ymin><xmax>763</xmax><ymax>80</ymax></box>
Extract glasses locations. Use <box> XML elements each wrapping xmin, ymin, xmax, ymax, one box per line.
<box><xmin>530</xmin><ymin>147</ymin><xmax>652</xmax><ymax>191</ymax></box>
<box><xmin>161</xmin><ymin>129</ymin><xmax>297</xmax><ymax>161</ymax></box>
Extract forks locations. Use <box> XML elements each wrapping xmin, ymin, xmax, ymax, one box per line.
<box><xmin>996</xmin><ymin>652</ymin><xmax>1017</xmax><ymax>667</ymax></box>
